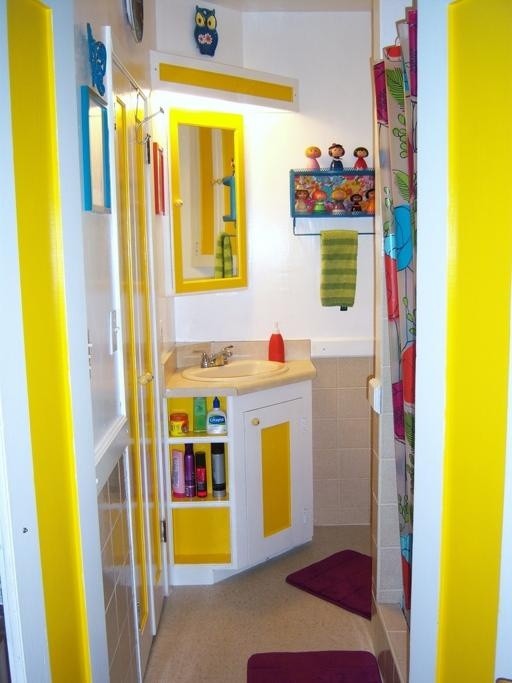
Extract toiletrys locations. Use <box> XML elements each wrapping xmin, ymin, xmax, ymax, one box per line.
<box><xmin>172</xmin><ymin>442</ymin><xmax>227</xmax><ymax>497</ymax></box>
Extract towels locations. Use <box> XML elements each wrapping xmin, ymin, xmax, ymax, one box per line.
<box><xmin>320</xmin><ymin>230</ymin><xmax>358</xmax><ymax>307</ymax></box>
<box><xmin>215</xmin><ymin>231</ymin><xmax>233</xmax><ymax>278</ymax></box>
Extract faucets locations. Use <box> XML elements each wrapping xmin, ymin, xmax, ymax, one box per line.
<box><xmin>210</xmin><ymin>350</ymin><xmax>232</xmax><ymax>366</ymax></box>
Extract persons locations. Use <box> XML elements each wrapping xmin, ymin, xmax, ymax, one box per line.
<box><xmin>295</xmin><ymin>142</ymin><xmax>374</xmax><ymax>216</ymax></box>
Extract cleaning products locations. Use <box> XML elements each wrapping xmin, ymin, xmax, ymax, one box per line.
<box><xmin>205</xmin><ymin>396</ymin><xmax>227</xmax><ymax>434</ymax></box>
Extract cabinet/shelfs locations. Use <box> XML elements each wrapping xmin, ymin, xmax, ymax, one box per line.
<box><xmin>244</xmin><ymin>397</ymin><xmax>312</xmax><ymax>565</ymax></box>
<box><xmin>162</xmin><ymin>389</ymin><xmax>237</xmax><ymax>569</ymax></box>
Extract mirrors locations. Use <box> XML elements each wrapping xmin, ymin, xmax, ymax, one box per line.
<box><xmin>168</xmin><ymin>106</ymin><xmax>247</xmax><ymax>293</ymax></box>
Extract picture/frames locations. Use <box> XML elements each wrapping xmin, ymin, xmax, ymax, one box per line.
<box><xmin>79</xmin><ymin>84</ymin><xmax>114</xmax><ymax>215</ymax></box>
<box><xmin>154</xmin><ymin>141</ymin><xmax>165</xmax><ymax>216</ymax></box>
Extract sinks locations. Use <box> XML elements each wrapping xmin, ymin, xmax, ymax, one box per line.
<box><xmin>179</xmin><ymin>358</ymin><xmax>287</xmax><ymax>381</ymax></box>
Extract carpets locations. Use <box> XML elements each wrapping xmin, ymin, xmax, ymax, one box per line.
<box><xmin>285</xmin><ymin>549</ymin><xmax>372</xmax><ymax>620</ymax></box>
<box><xmin>246</xmin><ymin>649</ymin><xmax>381</xmax><ymax>683</ymax></box>
